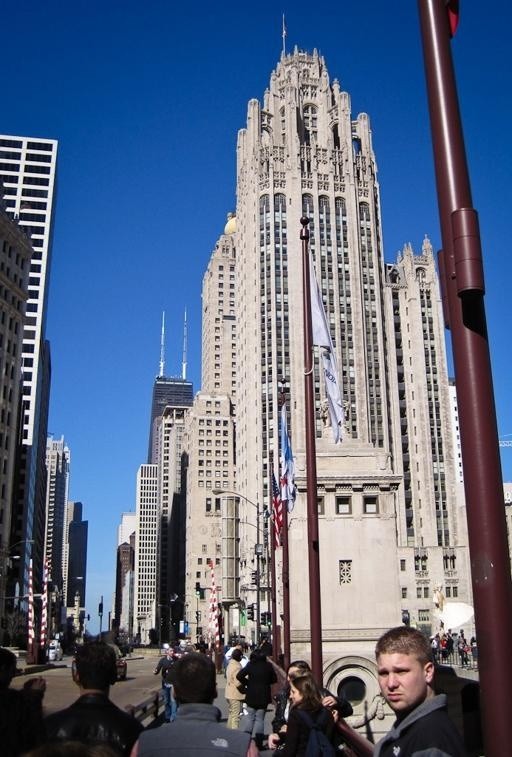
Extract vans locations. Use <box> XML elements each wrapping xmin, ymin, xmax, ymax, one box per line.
<box><xmin>48</xmin><ymin>640</ymin><xmax>63</xmax><ymax>661</ymax></box>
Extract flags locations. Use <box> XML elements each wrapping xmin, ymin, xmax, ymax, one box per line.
<box><xmin>276</xmin><ymin>402</ymin><xmax>299</xmax><ymax>516</ymax></box>
<box><xmin>262</xmin><ymin>454</ymin><xmax>283</xmax><ymax>553</ymax></box>
<box><xmin>307</xmin><ymin>240</ymin><xmax>349</xmax><ymax>445</ymax></box>
<box><xmin>444</xmin><ymin>0</ymin><xmax>460</xmax><ymax>40</ymax></box>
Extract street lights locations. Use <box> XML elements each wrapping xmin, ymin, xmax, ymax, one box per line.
<box><xmin>211</xmin><ymin>489</ymin><xmax>260</xmax><ymax>649</ymax></box>
<box><xmin>60</xmin><ymin>577</ymin><xmax>84</xmax><ymax>602</ymax></box>
<box><xmin>238</xmin><ymin>520</ymin><xmax>271</xmax><ymax>644</ymax></box>
<box><xmin>10</xmin><ymin>539</ymin><xmax>34</xmax><ymax>550</ymax></box>
<box><xmin>0</xmin><ymin>556</ymin><xmax>21</xmax><ymax>566</ymax></box>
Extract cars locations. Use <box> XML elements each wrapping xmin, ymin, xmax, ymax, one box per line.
<box><xmin>71</xmin><ymin>643</ymin><xmax>127</xmax><ymax>684</ymax></box>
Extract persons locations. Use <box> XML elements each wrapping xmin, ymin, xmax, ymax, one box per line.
<box><xmin>1</xmin><ymin>648</ymin><xmax>46</xmax><ymax>756</ymax></box>
<box><xmin>22</xmin><ymin>739</ymin><xmax>124</xmax><ymax>756</ymax></box>
<box><xmin>331</xmin><ymin>708</ymin><xmax>374</xmax><ymax>756</ymax></box>
<box><xmin>152</xmin><ymin>636</ymin><xmax>274</xmax><ymax>750</ymax></box>
<box><xmin>373</xmin><ymin>625</ymin><xmax>466</xmax><ymax>756</ymax></box>
<box><xmin>43</xmin><ymin>640</ymin><xmax>144</xmax><ymax>756</ymax></box>
<box><xmin>130</xmin><ymin>651</ymin><xmax>259</xmax><ymax>756</ymax></box>
<box><xmin>430</xmin><ymin>627</ymin><xmax>478</xmax><ymax>669</ymax></box>
<box><xmin>271</xmin><ymin>660</ymin><xmax>356</xmax><ymax>756</ymax></box>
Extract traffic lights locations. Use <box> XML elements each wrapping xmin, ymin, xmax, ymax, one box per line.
<box><xmin>246</xmin><ymin>603</ymin><xmax>254</xmax><ymax>621</ymax></box>
<box><xmin>267</xmin><ymin>612</ymin><xmax>271</xmax><ymax>626</ymax></box>
<box><xmin>260</xmin><ymin>612</ymin><xmax>267</xmax><ymax>626</ymax></box>
<box><xmin>195</xmin><ymin>582</ymin><xmax>200</xmax><ymax>595</ymax></box>
<box><xmin>98</xmin><ymin>604</ymin><xmax>103</xmax><ymax>616</ymax></box>
